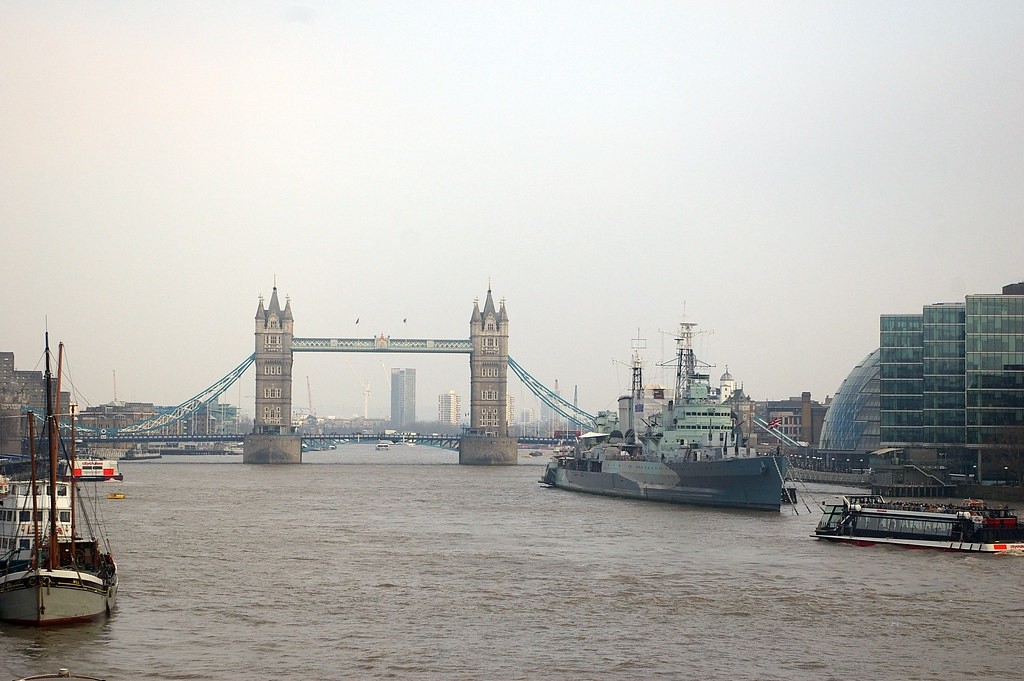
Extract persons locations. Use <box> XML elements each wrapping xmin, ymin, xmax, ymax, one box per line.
<box><xmin>860</xmin><ymin>499</ymin><xmax>1008</xmax><ymax>513</ymax></box>
<box><xmin>776</xmin><ymin>444</ymin><xmax>780</xmax><ymax>455</ymax></box>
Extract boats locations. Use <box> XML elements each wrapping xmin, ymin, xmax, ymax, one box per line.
<box><xmin>0</xmin><ymin>313</ymin><xmax>122</xmax><ymax>631</ymax></box>
<box><xmin>537</xmin><ymin>299</ymin><xmax>788</xmax><ymax>513</ymax></box>
<box><xmin>375</xmin><ymin>443</ymin><xmax>391</xmax><ymax>451</ymax></box>
<box><xmin>807</xmin><ymin>493</ymin><xmax>1023</xmax><ymax>557</ymax></box>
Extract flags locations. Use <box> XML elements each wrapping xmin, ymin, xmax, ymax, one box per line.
<box><xmin>765</xmin><ymin>418</ymin><xmax>781</xmax><ymax>429</ymax></box>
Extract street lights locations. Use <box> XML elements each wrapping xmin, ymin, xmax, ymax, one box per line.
<box><xmin>799</xmin><ymin>454</ymin><xmax>803</xmax><ymax>469</ymax></box>
<box><xmin>832</xmin><ymin>458</ymin><xmax>835</xmax><ymax>472</ymax></box>
<box><xmin>1004</xmin><ymin>466</ymin><xmax>1008</xmax><ymax>486</ymax></box>
<box><xmin>817</xmin><ymin>457</ymin><xmax>820</xmax><ymax>470</ymax></box>
<box><xmin>859</xmin><ymin>459</ymin><xmax>863</xmax><ymax>475</ymax></box>
<box><xmin>790</xmin><ymin>454</ymin><xmax>793</xmax><ymax>467</ymax></box>
<box><xmin>812</xmin><ymin>455</ymin><xmax>816</xmax><ymax>472</ymax></box>
<box><xmin>846</xmin><ymin>458</ymin><xmax>850</xmax><ymax>474</ymax></box>
<box><xmin>820</xmin><ymin>457</ymin><xmax>823</xmax><ymax>471</ymax></box>
<box><xmin>794</xmin><ymin>454</ymin><xmax>797</xmax><ymax>470</ymax></box>
<box><xmin>973</xmin><ymin>465</ymin><xmax>977</xmax><ymax>481</ymax></box>
<box><xmin>806</xmin><ymin>456</ymin><xmax>809</xmax><ymax>470</ymax></box>
